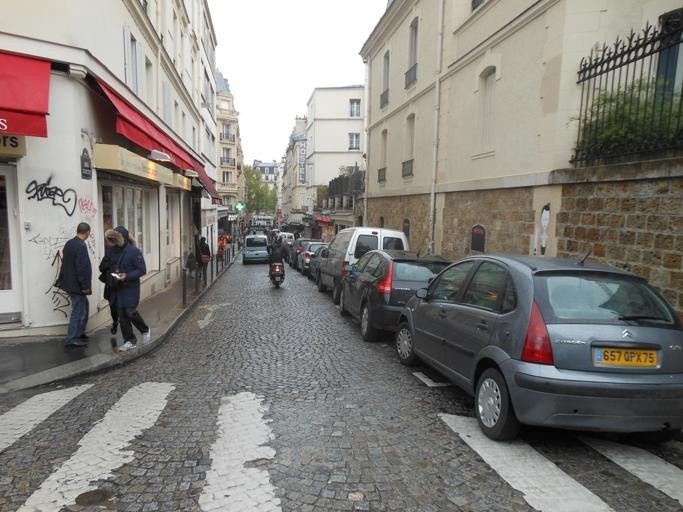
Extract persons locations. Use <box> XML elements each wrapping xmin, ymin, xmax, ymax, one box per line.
<box><xmin>218</xmin><ymin>235</ymin><xmax>225</xmax><ymax>262</ymax></box>
<box><xmin>104</xmin><ymin>227</ymin><xmax>152</xmax><ymax>350</ymax></box>
<box><xmin>537</xmin><ymin>201</ymin><xmax>551</xmax><ymax>256</ymax></box>
<box><xmin>265</xmin><ymin>238</ymin><xmax>288</xmax><ymax>272</ymax></box>
<box><xmin>98</xmin><ymin>235</ymin><xmax>135</xmax><ymax>334</ymax></box>
<box><xmin>198</xmin><ymin>237</ymin><xmax>209</xmax><ymax>289</ymax></box>
<box><xmin>55</xmin><ymin>220</ymin><xmax>93</xmax><ymax>347</ymax></box>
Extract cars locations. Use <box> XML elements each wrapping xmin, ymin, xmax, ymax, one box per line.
<box><xmin>239</xmin><ymin>233</ymin><xmax>273</xmax><ymax>263</ymax></box>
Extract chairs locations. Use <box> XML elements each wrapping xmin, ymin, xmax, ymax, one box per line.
<box><xmin>551</xmin><ymin>284</ymin><xmax>593</xmax><ymax>310</ymax></box>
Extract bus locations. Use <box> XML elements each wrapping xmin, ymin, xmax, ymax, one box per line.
<box><xmin>249</xmin><ymin>211</ymin><xmax>276</xmax><ymax>229</ymax></box>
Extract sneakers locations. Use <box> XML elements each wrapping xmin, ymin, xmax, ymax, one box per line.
<box><xmin>140</xmin><ymin>326</ymin><xmax>151</xmax><ymax>347</ymax></box>
<box><xmin>110</xmin><ymin>321</ymin><xmax>118</xmax><ymax>335</ymax></box>
<box><xmin>118</xmin><ymin>340</ymin><xmax>137</xmax><ymax>352</ymax></box>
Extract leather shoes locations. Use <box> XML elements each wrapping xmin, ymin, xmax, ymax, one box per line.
<box><xmin>81</xmin><ymin>334</ymin><xmax>90</xmax><ymax>340</ymax></box>
<box><xmin>65</xmin><ymin>340</ymin><xmax>88</xmax><ymax>348</ymax></box>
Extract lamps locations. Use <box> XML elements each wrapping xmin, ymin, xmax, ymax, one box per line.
<box><xmin>182</xmin><ymin>169</ymin><xmax>199</xmax><ymax>177</ymax></box>
<box><xmin>148</xmin><ymin>150</ymin><xmax>170</xmax><ymax>161</ymax></box>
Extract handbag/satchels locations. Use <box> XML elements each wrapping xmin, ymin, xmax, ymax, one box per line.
<box><xmin>200</xmin><ymin>254</ymin><xmax>210</xmax><ymax>263</ymax></box>
<box><xmin>97</xmin><ymin>271</ymin><xmax>107</xmax><ymax>283</ymax></box>
<box><xmin>53</xmin><ymin>263</ymin><xmax>64</xmax><ymax>289</ymax></box>
